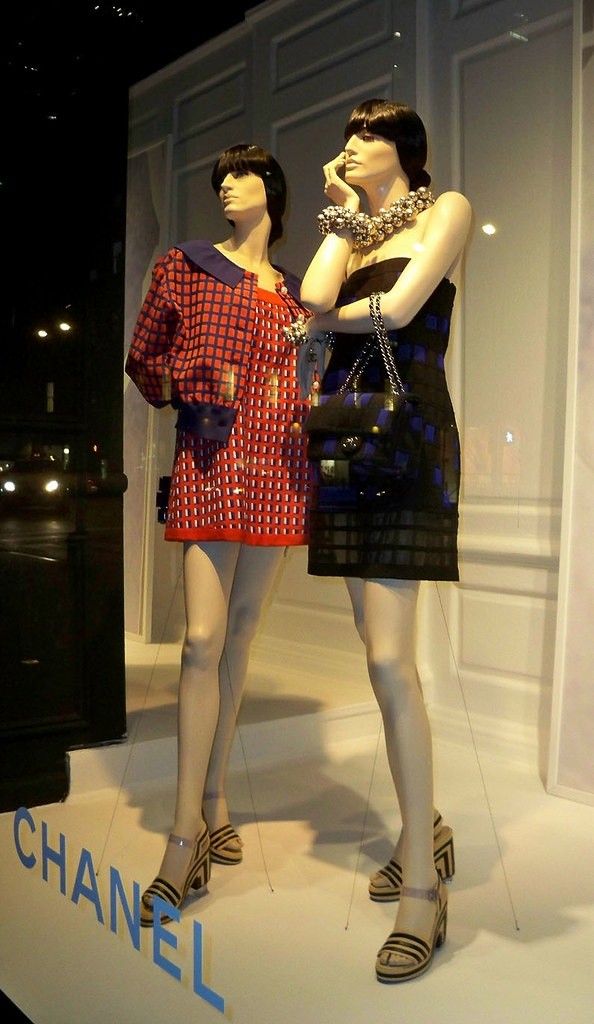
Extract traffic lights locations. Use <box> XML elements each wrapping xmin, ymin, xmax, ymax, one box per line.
<box><xmin>88</xmin><ymin>442</ymin><xmax>101</xmax><ymax>456</ymax></box>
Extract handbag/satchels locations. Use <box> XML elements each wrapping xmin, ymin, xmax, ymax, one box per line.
<box><xmin>302</xmin><ymin>292</ymin><xmax>428</xmax><ymax>508</ymax></box>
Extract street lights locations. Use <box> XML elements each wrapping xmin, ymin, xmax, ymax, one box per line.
<box><xmin>33</xmin><ymin>302</ymin><xmax>86</xmax><ymax>426</ymax></box>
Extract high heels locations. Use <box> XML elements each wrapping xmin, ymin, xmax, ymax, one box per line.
<box><xmin>368</xmin><ymin>807</ymin><xmax>454</xmax><ymax>902</ymax></box>
<box><xmin>375</xmin><ymin>868</ymin><xmax>449</xmax><ymax>983</ymax></box>
<box><xmin>139</xmin><ymin>819</ymin><xmax>211</xmax><ymax>927</ymax></box>
<box><xmin>201</xmin><ymin>791</ymin><xmax>243</xmax><ymax>864</ymax></box>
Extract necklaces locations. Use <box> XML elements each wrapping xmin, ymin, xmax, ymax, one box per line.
<box><xmin>352</xmin><ymin>186</ymin><xmax>436</xmax><ymax>251</ymax></box>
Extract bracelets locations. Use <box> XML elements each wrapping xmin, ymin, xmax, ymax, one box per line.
<box><xmin>316</xmin><ymin>205</ymin><xmax>368</xmax><ymax>241</ymax></box>
<box><xmin>278</xmin><ymin>316</ymin><xmax>336</xmax><ymax>364</ymax></box>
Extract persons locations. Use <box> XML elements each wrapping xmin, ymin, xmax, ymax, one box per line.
<box><xmin>296</xmin><ymin>97</ymin><xmax>473</xmax><ymax>984</ymax></box>
<box><xmin>125</xmin><ymin>141</ymin><xmax>316</xmax><ymax>928</ymax></box>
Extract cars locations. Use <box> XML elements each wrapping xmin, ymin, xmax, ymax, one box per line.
<box><xmin>0</xmin><ymin>453</ymin><xmax>65</xmax><ymax>511</ymax></box>
